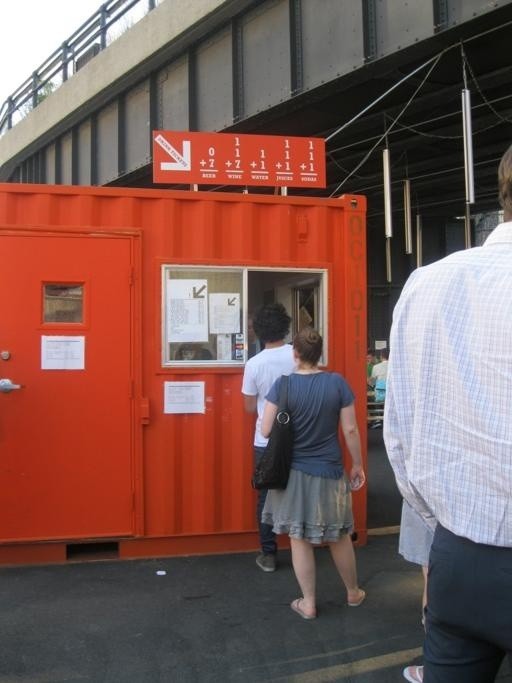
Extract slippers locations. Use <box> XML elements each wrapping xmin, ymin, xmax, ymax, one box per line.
<box><xmin>291</xmin><ymin>597</ymin><xmax>316</xmax><ymax>619</ymax></box>
<box><xmin>348</xmin><ymin>588</ymin><xmax>366</xmax><ymax>605</ymax></box>
<box><xmin>403</xmin><ymin>665</ymin><xmax>423</xmax><ymax>683</ymax></box>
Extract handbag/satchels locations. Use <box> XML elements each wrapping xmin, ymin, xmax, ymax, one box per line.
<box><xmin>253</xmin><ymin>375</ymin><xmax>295</xmax><ymax>490</ymax></box>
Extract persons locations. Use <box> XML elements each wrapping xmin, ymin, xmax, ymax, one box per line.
<box><xmin>368</xmin><ymin>347</ymin><xmax>378</xmax><ymax>378</ymax></box>
<box><xmin>398</xmin><ymin>500</ymin><xmax>434</xmax><ymax>683</ymax></box>
<box><xmin>368</xmin><ymin>347</ymin><xmax>388</xmax><ymax>430</ymax></box>
<box><xmin>260</xmin><ymin>329</ymin><xmax>368</xmax><ymax>621</ymax></box>
<box><xmin>241</xmin><ymin>301</ymin><xmax>301</xmax><ymax>574</ymax></box>
<box><xmin>381</xmin><ymin>144</ymin><xmax>511</xmax><ymax>683</ymax></box>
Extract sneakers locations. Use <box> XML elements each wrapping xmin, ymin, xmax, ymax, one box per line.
<box><xmin>256</xmin><ymin>554</ymin><xmax>275</xmax><ymax>571</ymax></box>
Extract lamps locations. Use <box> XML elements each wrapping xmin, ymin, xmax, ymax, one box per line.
<box><xmin>459</xmin><ymin>57</ymin><xmax>478</xmax><ymax>206</ymax></box>
<box><xmin>380</xmin><ymin>138</ymin><xmax>423</xmax><ymax>281</ymax></box>
